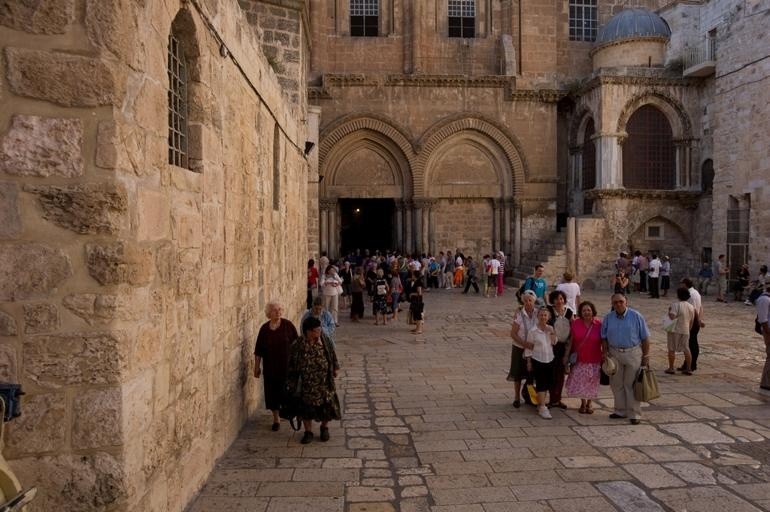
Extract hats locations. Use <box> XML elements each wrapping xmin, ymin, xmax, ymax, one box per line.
<box><xmin>660</xmin><ymin>255</ymin><xmax>671</xmax><ymax>260</ymax></box>
<box><xmin>601</xmin><ymin>356</ymin><xmax>619</xmax><ymax>376</ymax></box>
<box><xmin>622</xmin><ymin>251</ymin><xmax>629</xmax><ymax>255</ymax></box>
<box><xmin>742</xmin><ymin>263</ymin><xmax>749</xmax><ymax>270</ymax></box>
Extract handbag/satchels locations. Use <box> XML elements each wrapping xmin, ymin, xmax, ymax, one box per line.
<box><xmin>522</xmin><ymin>379</ymin><xmax>547</xmax><ymax>405</ymax></box>
<box><xmin>754</xmin><ymin>313</ymin><xmax>764</xmax><ymax>335</ymax></box>
<box><xmin>515</xmin><ymin>277</ymin><xmax>535</xmax><ymax>306</ymax></box>
<box><xmin>411</xmin><ymin>295</ymin><xmax>424</xmax><ymax>314</ymax></box>
<box><xmin>599</xmin><ymin>367</ymin><xmax>610</xmax><ymax>386</ymax></box>
<box><xmin>335</xmin><ymin>267</ymin><xmax>427</xmax><ymax>296</ymax></box>
<box><xmin>663</xmin><ymin>302</ymin><xmax>680</xmax><ymax>334</ymax></box>
<box><xmin>633</xmin><ymin>366</ymin><xmax>661</xmax><ymax>402</ymax></box>
<box><xmin>563</xmin><ymin>352</ymin><xmax>577</xmax><ymax>374</ymax></box>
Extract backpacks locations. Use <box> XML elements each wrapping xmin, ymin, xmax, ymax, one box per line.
<box><xmin>277</xmin><ymin>369</ymin><xmax>303</xmax><ymax>430</ymax></box>
<box><xmin>552</xmin><ymin>307</ymin><xmax>571</xmax><ymax>344</ymax></box>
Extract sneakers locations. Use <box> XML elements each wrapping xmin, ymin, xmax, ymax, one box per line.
<box><xmin>423</xmin><ymin>283</ymin><xmax>463</xmax><ymax>293</ymax></box>
<box><xmin>319</xmin><ymin>425</ymin><xmax>330</xmax><ymax>441</ymax></box>
<box><xmin>350</xmin><ymin>308</ymin><xmax>401</xmax><ymax>326</ymax></box>
<box><xmin>759</xmin><ymin>384</ymin><xmax>770</xmax><ymax>390</ymax></box>
<box><xmin>639</xmin><ymin>290</ymin><xmax>668</xmax><ymax>299</ymax></box>
<box><xmin>717</xmin><ymin>296</ymin><xmax>754</xmax><ymax>306</ymax></box>
<box><xmin>473</xmin><ymin>291</ymin><xmax>504</xmax><ymax>297</ymax></box>
<box><xmin>300</xmin><ymin>431</ymin><xmax>314</xmax><ymax>444</ymax></box>
<box><xmin>460</xmin><ymin>291</ymin><xmax>467</xmax><ymax>294</ymax></box>
<box><xmin>537</xmin><ymin>405</ymin><xmax>553</xmax><ymax>419</ymax></box>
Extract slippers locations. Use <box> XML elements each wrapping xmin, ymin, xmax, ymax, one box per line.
<box><xmin>664</xmin><ymin>368</ymin><xmax>675</xmax><ymax>373</ymax></box>
<box><xmin>682</xmin><ymin>369</ymin><xmax>692</xmax><ymax>376</ymax></box>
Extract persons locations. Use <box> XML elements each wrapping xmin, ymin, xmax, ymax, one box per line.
<box><xmin>614</xmin><ymin>248</ymin><xmax>769</xmax><ymax>306</ymax></box>
<box><xmin>284</xmin><ymin>317</ymin><xmax>341</xmax><ymax>444</ymax></box>
<box><xmin>663</xmin><ymin>287</ymin><xmax>697</xmax><ymax>376</ymax></box>
<box><xmin>300</xmin><ymin>297</ymin><xmax>339</xmax><ymax>350</ymax></box>
<box><xmin>252</xmin><ymin>300</ymin><xmax>299</xmax><ymax>432</ymax></box>
<box><xmin>677</xmin><ymin>277</ymin><xmax>706</xmax><ymax>371</ymax></box>
<box><xmin>755</xmin><ymin>287</ymin><xmax>770</xmax><ymax>389</ymax></box>
<box><xmin>307</xmin><ymin>244</ymin><xmax>506</xmax><ymax>335</ymax></box>
<box><xmin>505</xmin><ymin>264</ymin><xmax>651</xmax><ymax>424</ymax></box>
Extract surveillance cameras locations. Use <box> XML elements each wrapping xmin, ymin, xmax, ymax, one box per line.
<box><xmin>352</xmin><ymin>206</ymin><xmax>362</xmax><ymax>215</ymax></box>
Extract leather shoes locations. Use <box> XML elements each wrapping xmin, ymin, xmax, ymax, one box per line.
<box><xmin>550</xmin><ymin>400</ymin><xmax>567</xmax><ymax>409</ymax></box>
<box><xmin>409</xmin><ymin>329</ymin><xmax>424</xmax><ymax>334</ymax></box>
<box><xmin>513</xmin><ymin>399</ymin><xmax>520</xmax><ymax>408</ymax></box>
<box><xmin>630</xmin><ymin>416</ymin><xmax>640</xmax><ymax>424</ymax></box>
<box><xmin>609</xmin><ymin>412</ymin><xmax>626</xmax><ymax>419</ymax></box>
<box><xmin>676</xmin><ymin>362</ymin><xmax>697</xmax><ymax>372</ymax></box>
<box><xmin>272</xmin><ymin>417</ymin><xmax>280</xmax><ymax>431</ymax></box>
<box><xmin>578</xmin><ymin>403</ymin><xmax>594</xmax><ymax>414</ymax></box>
